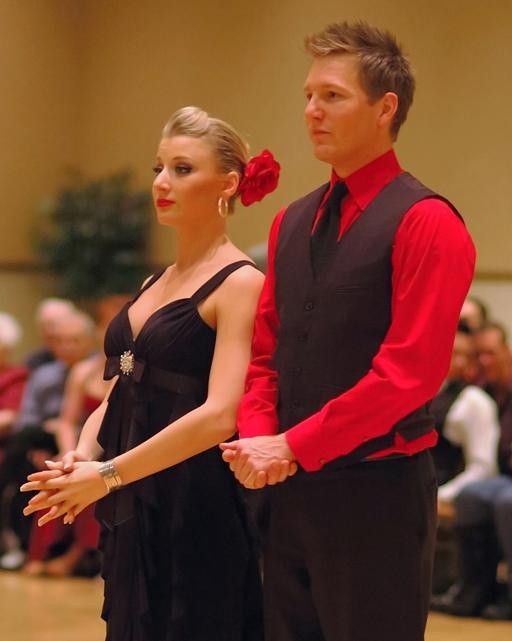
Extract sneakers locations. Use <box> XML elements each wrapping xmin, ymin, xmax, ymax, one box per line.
<box><xmin>1</xmin><ymin>548</ymin><xmax>101</xmax><ymax>582</ymax></box>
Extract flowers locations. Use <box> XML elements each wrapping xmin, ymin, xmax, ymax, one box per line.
<box><xmin>236</xmin><ymin>148</ymin><xmax>281</xmax><ymax>207</ymax></box>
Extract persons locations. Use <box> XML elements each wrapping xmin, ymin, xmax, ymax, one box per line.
<box><xmin>0</xmin><ymin>295</ymin><xmax>511</xmax><ymax>619</ymax></box>
<box><xmin>219</xmin><ymin>20</ymin><xmax>476</xmax><ymax>641</ymax></box>
<box><xmin>18</xmin><ymin>106</ymin><xmax>279</xmax><ymax>641</ymax></box>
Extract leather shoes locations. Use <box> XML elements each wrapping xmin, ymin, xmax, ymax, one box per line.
<box><xmin>429</xmin><ymin>580</ymin><xmax>512</xmax><ymax>621</ymax></box>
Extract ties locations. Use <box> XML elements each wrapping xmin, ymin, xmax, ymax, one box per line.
<box><xmin>311</xmin><ymin>182</ymin><xmax>352</xmax><ymax>278</ymax></box>
